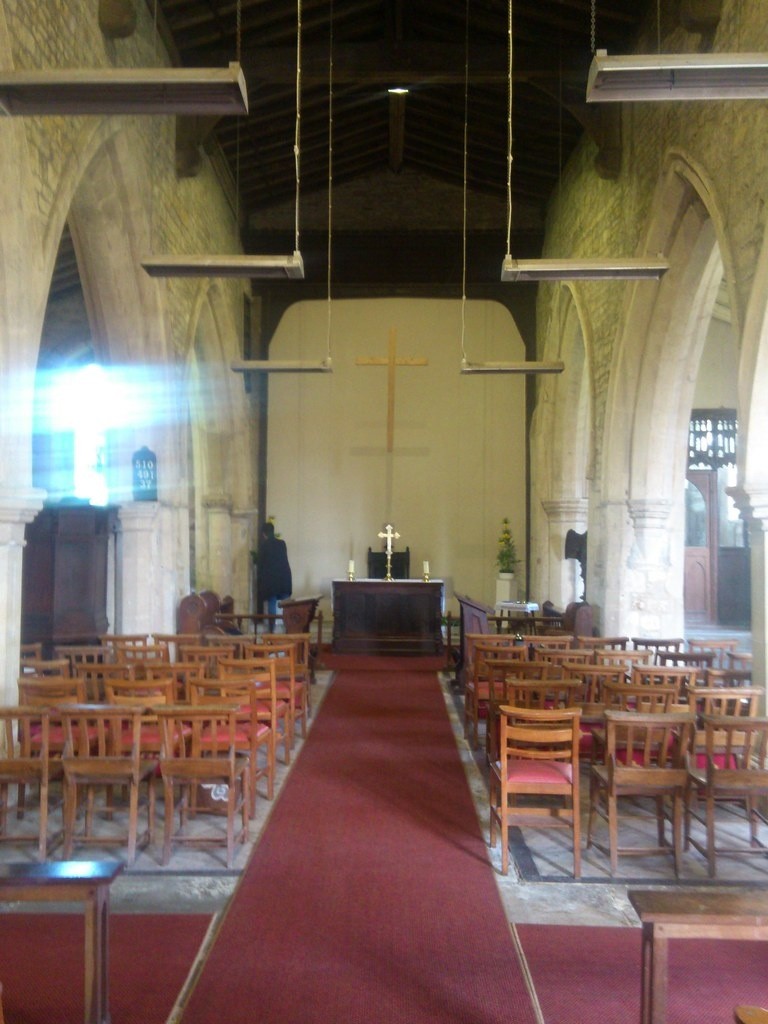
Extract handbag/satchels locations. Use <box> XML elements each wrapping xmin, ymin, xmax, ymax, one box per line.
<box><xmin>197</xmin><ymin>776</ymin><xmax>242</xmax><ymax>815</ymax></box>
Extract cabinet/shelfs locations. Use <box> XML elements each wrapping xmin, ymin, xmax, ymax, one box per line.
<box><xmin>333</xmin><ymin>581</ymin><xmax>444</xmax><ymax>656</ymax></box>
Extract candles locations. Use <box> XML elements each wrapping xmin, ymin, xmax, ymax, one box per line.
<box><xmin>423</xmin><ymin>560</ymin><xmax>429</xmax><ymax>573</ymax></box>
<box><xmin>348</xmin><ymin>559</ymin><xmax>354</xmax><ymax>572</ymax></box>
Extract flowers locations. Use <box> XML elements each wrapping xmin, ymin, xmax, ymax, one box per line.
<box><xmin>496</xmin><ymin>517</ymin><xmax>522</xmax><ymax>573</ymax></box>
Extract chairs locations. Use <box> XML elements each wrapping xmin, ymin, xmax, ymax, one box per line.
<box><xmin>465</xmin><ymin>631</ymin><xmax>768</xmax><ymax>883</ymax></box>
<box><xmin>0</xmin><ymin>632</ymin><xmax>313</xmax><ymax>862</ymax></box>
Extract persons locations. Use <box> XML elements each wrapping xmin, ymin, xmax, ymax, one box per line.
<box><xmin>250</xmin><ymin>522</ymin><xmax>293</xmax><ymax>633</ymax></box>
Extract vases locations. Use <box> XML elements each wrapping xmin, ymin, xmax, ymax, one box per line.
<box><xmin>499</xmin><ymin>573</ymin><xmax>515</xmax><ymax>580</ymax></box>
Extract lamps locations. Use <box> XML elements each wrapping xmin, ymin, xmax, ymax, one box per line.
<box><xmin>460</xmin><ymin>0</ymin><xmax>768</xmax><ymax>374</ymax></box>
<box><xmin>0</xmin><ymin>0</ymin><xmax>333</xmax><ymax>371</ymax></box>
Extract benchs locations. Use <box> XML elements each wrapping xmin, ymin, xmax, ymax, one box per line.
<box><xmin>0</xmin><ymin>862</ymin><xmax>126</xmax><ymax>1024</ymax></box>
<box><xmin>628</xmin><ymin>888</ymin><xmax>768</xmax><ymax>1024</ymax></box>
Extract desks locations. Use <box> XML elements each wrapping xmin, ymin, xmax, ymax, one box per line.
<box><xmin>496</xmin><ymin>601</ymin><xmax>539</xmax><ymax>635</ymax></box>
